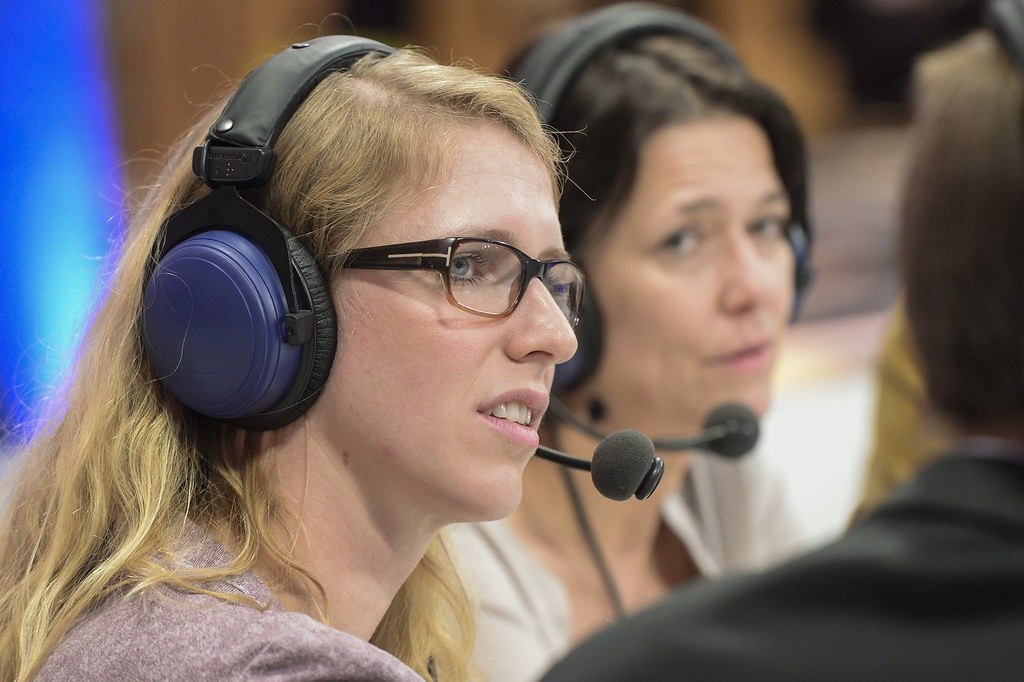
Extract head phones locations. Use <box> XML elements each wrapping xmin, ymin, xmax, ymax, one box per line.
<box><xmin>507</xmin><ymin>4</ymin><xmax>814</xmax><ymax>400</ymax></box>
<box><xmin>137</xmin><ymin>33</ymin><xmax>399</xmax><ymax>432</ymax></box>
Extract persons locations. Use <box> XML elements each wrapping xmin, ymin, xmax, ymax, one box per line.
<box><xmin>422</xmin><ymin>6</ymin><xmax>848</xmax><ymax>682</ymax></box>
<box><xmin>0</xmin><ymin>35</ymin><xmax>581</xmax><ymax>682</ymax></box>
<box><xmin>533</xmin><ymin>61</ymin><xmax>1022</xmax><ymax>682</ymax></box>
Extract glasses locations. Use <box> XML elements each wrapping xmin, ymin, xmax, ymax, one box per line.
<box><xmin>319</xmin><ymin>235</ymin><xmax>587</xmax><ymax>332</ymax></box>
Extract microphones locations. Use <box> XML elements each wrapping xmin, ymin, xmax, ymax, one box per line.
<box><xmin>534</xmin><ymin>390</ymin><xmax>759</xmax><ymax>501</ymax></box>
